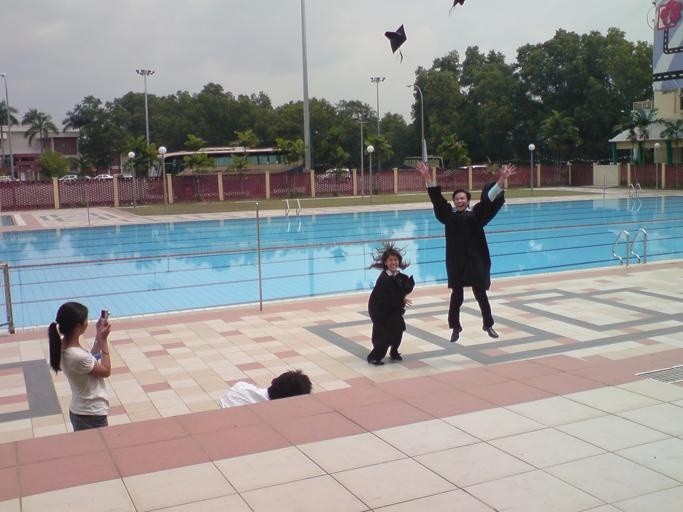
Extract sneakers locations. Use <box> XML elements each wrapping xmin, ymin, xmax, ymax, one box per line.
<box><xmin>366</xmin><ymin>356</ymin><xmax>384</xmax><ymax>365</ymax></box>
<box><xmin>390</xmin><ymin>353</ymin><xmax>402</xmax><ymax>360</ymax></box>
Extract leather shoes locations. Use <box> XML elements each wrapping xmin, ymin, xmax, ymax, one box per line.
<box><xmin>483</xmin><ymin>324</ymin><xmax>499</xmax><ymax>338</ymax></box>
<box><xmin>451</xmin><ymin>327</ymin><xmax>462</xmax><ymax>341</ymax></box>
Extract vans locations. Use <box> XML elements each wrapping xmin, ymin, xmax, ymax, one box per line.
<box><xmin>456</xmin><ymin>163</ymin><xmax>490</xmax><ymax>185</ymax></box>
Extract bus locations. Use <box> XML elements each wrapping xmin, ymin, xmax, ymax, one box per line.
<box><xmin>397</xmin><ymin>155</ymin><xmax>446</xmax><ymax>184</ymax></box>
<box><xmin>145</xmin><ymin>146</ymin><xmax>302</xmax><ymax>199</ymax></box>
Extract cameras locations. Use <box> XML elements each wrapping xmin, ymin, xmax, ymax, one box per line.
<box><xmin>101</xmin><ymin>309</ymin><xmax>110</xmax><ymax>318</ymax></box>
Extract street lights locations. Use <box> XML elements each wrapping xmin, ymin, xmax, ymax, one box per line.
<box><xmin>369</xmin><ymin>75</ymin><xmax>385</xmax><ymax>171</ymax></box>
<box><xmin>0</xmin><ymin>73</ymin><xmax>14</xmax><ymax>182</ymax></box>
<box><xmin>404</xmin><ymin>83</ymin><xmax>427</xmax><ymax>167</ymax></box>
<box><xmin>135</xmin><ymin>68</ymin><xmax>156</xmax><ymax>177</ymax></box>
<box><xmin>352</xmin><ymin>107</ymin><xmax>371</xmax><ymax>201</ymax></box>
<box><xmin>527</xmin><ymin>143</ymin><xmax>535</xmax><ymax>195</ymax></box>
<box><xmin>366</xmin><ymin>144</ymin><xmax>376</xmax><ymax>197</ymax></box>
<box><xmin>527</xmin><ymin>204</ymin><xmax>536</xmax><ymax>248</ymax></box>
<box><xmin>133</xmin><ymin>223</ymin><xmax>178</xmax><ymax>295</ymax></box>
<box><xmin>355</xmin><ymin>213</ymin><xmax>377</xmax><ymax>291</ymax></box>
<box><xmin>157</xmin><ymin>145</ymin><xmax>169</xmax><ymax>209</ymax></box>
<box><xmin>127</xmin><ymin>151</ymin><xmax>137</xmax><ymax>210</ymax></box>
<box><xmin>652</xmin><ymin>140</ymin><xmax>662</xmax><ymax>192</ymax></box>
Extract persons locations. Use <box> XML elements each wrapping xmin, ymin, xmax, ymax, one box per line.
<box><xmin>215</xmin><ymin>368</ymin><xmax>312</xmax><ymax>411</ymax></box>
<box><xmin>367</xmin><ymin>238</ymin><xmax>416</xmax><ymax>365</ymax></box>
<box><xmin>415</xmin><ymin>158</ymin><xmax>517</xmax><ymax>342</ymax></box>
<box><xmin>48</xmin><ymin>301</ymin><xmax>112</xmax><ymax>430</ymax></box>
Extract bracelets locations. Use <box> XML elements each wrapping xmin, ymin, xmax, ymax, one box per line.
<box><xmin>102</xmin><ymin>351</ymin><xmax>109</xmax><ymax>355</ymax></box>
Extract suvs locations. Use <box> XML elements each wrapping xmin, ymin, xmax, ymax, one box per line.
<box><xmin>317</xmin><ymin>166</ymin><xmax>352</xmax><ymax>186</ymax></box>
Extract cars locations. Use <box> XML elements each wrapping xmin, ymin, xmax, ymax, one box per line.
<box><xmin>0</xmin><ymin>174</ymin><xmax>18</xmax><ymax>187</ymax></box>
<box><xmin>57</xmin><ymin>170</ymin><xmax>113</xmax><ymax>183</ymax></box>
